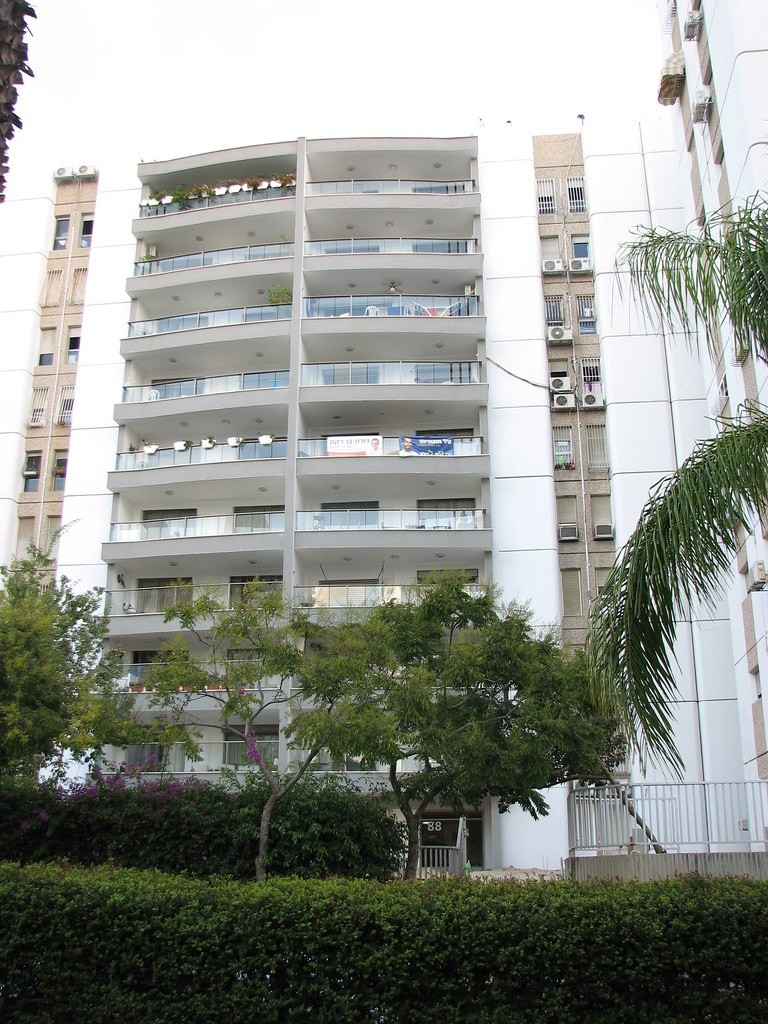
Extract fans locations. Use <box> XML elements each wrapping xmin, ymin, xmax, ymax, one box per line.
<box><xmin>382</xmin><ymin>281</ymin><xmax>404</xmax><ymax>293</ymax></box>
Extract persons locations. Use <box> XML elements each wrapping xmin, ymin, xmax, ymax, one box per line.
<box><xmin>367</xmin><ymin>439</ymin><xmax>382</xmax><ymax>455</ymax></box>
<box><xmin>399</xmin><ymin>437</ymin><xmax>419</xmax><ymax>455</ymax></box>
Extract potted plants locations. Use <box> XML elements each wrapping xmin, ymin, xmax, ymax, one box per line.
<box><xmin>128</xmin><ymin>680</ymin><xmax>218</xmax><ymax>693</ymax></box>
<box><xmin>256</xmin><ymin>431</ymin><xmax>274</xmax><ymax>445</ymax></box>
<box><xmin>201</xmin><ymin>435</ymin><xmax>216</xmax><ymax>449</ymax></box>
<box><xmin>227</xmin><ymin>436</ymin><xmax>244</xmax><ymax>447</ymax></box>
<box><xmin>147</xmin><ymin>171</ymin><xmax>296</xmax><ymax>211</ymax></box>
<box><xmin>174</xmin><ymin>439</ymin><xmax>193</xmax><ymax>450</ymax></box>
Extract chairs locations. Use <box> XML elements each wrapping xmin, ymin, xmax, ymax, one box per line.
<box><xmin>364</xmin><ymin>305</ymin><xmax>379</xmax><ymax>315</ymax></box>
<box><xmin>148</xmin><ymin>390</ymin><xmax>160</xmax><ymax>400</ymax></box>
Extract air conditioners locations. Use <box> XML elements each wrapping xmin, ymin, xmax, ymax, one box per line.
<box><xmin>581</xmin><ymin>394</ymin><xmax>604</xmax><ymax>408</ymax></box>
<box><xmin>594</xmin><ymin>524</ymin><xmax>614</xmax><ymax>539</ymax></box>
<box><xmin>548</xmin><ymin>326</ymin><xmax>572</xmax><ymax>345</ymax></box>
<box><xmin>542</xmin><ymin>260</ymin><xmax>564</xmax><ymax>275</ymax></box>
<box><xmin>569</xmin><ymin>258</ymin><xmax>590</xmax><ymax>276</ymax></box>
<box><xmin>691</xmin><ymin>89</ymin><xmax>705</xmax><ymax>124</ymax></box>
<box><xmin>54</xmin><ymin>166</ymin><xmax>74</xmax><ymax>181</ymax></box>
<box><xmin>29</xmin><ymin>416</ymin><xmax>45</xmax><ymax>427</ymax></box>
<box><xmin>683</xmin><ymin>10</ymin><xmax>696</xmax><ymax>40</ymax></box>
<box><xmin>553</xmin><ymin>394</ymin><xmax>576</xmax><ymax>411</ymax></box>
<box><xmin>549</xmin><ymin>377</ymin><xmax>570</xmax><ymax>393</ymax></box>
<box><xmin>558</xmin><ymin>524</ymin><xmax>579</xmax><ymax>542</ymax></box>
<box><xmin>74</xmin><ymin>165</ymin><xmax>96</xmax><ymax>179</ymax></box>
